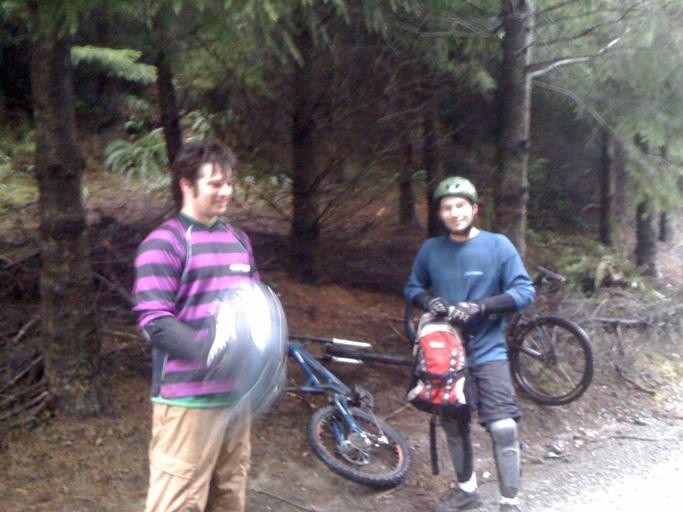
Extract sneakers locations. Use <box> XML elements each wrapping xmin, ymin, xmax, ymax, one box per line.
<box><xmin>498</xmin><ymin>503</ymin><xmax>522</xmax><ymax>512</ymax></box>
<box><xmin>434</xmin><ymin>479</ymin><xmax>481</xmax><ymax>512</ymax></box>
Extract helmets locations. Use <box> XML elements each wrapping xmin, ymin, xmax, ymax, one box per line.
<box><xmin>429</xmin><ymin>174</ymin><xmax>481</xmax><ymax>211</ymax></box>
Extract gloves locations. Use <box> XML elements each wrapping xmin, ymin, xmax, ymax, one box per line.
<box><xmin>426</xmin><ymin>297</ymin><xmax>452</xmax><ymax>320</ymax></box>
<box><xmin>450</xmin><ymin>299</ymin><xmax>486</xmax><ymax>324</ymax></box>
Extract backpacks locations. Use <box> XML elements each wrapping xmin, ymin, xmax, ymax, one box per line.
<box><xmin>403</xmin><ymin>306</ymin><xmax>473</xmax><ymax>418</ymax></box>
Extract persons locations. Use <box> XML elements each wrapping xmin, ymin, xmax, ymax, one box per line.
<box><xmin>399</xmin><ymin>175</ymin><xmax>537</xmax><ymax>511</ymax></box>
<box><xmin>128</xmin><ymin>135</ymin><xmax>262</xmax><ymax>512</ymax></box>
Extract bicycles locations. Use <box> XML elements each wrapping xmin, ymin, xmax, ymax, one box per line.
<box><xmin>402</xmin><ymin>261</ymin><xmax>595</xmax><ymax>406</ymax></box>
<box><xmin>279</xmin><ymin>327</ymin><xmax>416</xmax><ymax>488</ymax></box>
